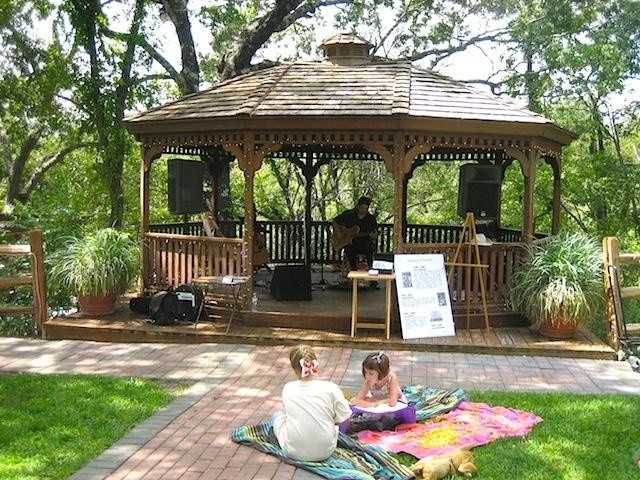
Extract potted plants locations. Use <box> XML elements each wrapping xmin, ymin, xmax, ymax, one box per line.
<box><xmin>503</xmin><ymin>229</ymin><xmax>619</xmax><ymax>340</ymax></box>
<box><xmin>43</xmin><ymin>218</ymin><xmax>151</xmax><ymax>317</ymax></box>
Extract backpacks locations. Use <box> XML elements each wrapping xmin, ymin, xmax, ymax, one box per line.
<box><xmin>148</xmin><ymin>285</ymin><xmax>178</xmax><ymax>326</ymax></box>
<box><xmin>174</xmin><ymin>280</ymin><xmax>205</xmax><ymax>321</ymax></box>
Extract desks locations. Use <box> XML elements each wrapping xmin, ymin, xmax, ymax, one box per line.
<box><xmin>346</xmin><ymin>270</ymin><xmax>395</xmax><ymax>340</ymax></box>
<box><xmin>189</xmin><ymin>273</ymin><xmax>253</xmax><ymax>334</ymax></box>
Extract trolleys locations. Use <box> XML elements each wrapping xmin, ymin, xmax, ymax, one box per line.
<box><xmin>607</xmin><ymin>265</ymin><xmax>640</xmax><ymax>374</ymax></box>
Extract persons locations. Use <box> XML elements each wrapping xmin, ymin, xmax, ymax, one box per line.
<box><xmin>333</xmin><ymin>196</ymin><xmax>380</xmax><ymax>289</ymax></box>
<box><xmin>350</xmin><ymin>352</ymin><xmax>407</xmax><ymax>412</ymax></box>
<box><xmin>273</xmin><ymin>346</ymin><xmax>352</xmax><ymax>462</ymax></box>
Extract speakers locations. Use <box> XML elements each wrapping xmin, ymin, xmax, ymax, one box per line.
<box><xmin>167</xmin><ymin>159</ymin><xmax>204</xmax><ymax>215</ymax></box>
<box><xmin>457</xmin><ymin>163</ymin><xmax>503</xmax><ymax>220</ymax></box>
<box><xmin>270</xmin><ymin>264</ymin><xmax>313</xmax><ymax>301</ymax></box>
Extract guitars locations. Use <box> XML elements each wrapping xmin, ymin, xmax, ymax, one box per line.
<box><xmin>333</xmin><ymin>225</ymin><xmax>384</xmax><ymax>251</ymax></box>
<box><xmin>253</xmin><ymin>203</ymin><xmax>269</xmax><ymax>266</ymax></box>
